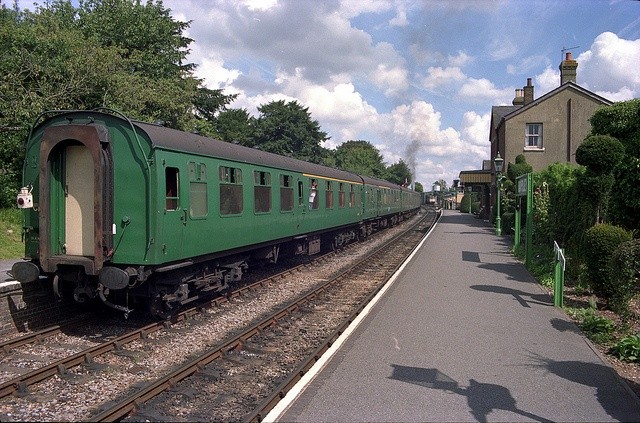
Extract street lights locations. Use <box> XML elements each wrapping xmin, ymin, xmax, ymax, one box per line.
<box><xmin>467</xmin><ymin>185</ymin><xmax>473</xmax><ymax>214</ymax></box>
<box><xmin>492</xmin><ymin>151</ymin><xmax>505</xmax><ymax>236</ymax></box>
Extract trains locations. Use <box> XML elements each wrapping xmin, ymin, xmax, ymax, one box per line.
<box><xmin>6</xmin><ymin>107</ymin><xmax>422</xmax><ymax>322</ymax></box>
